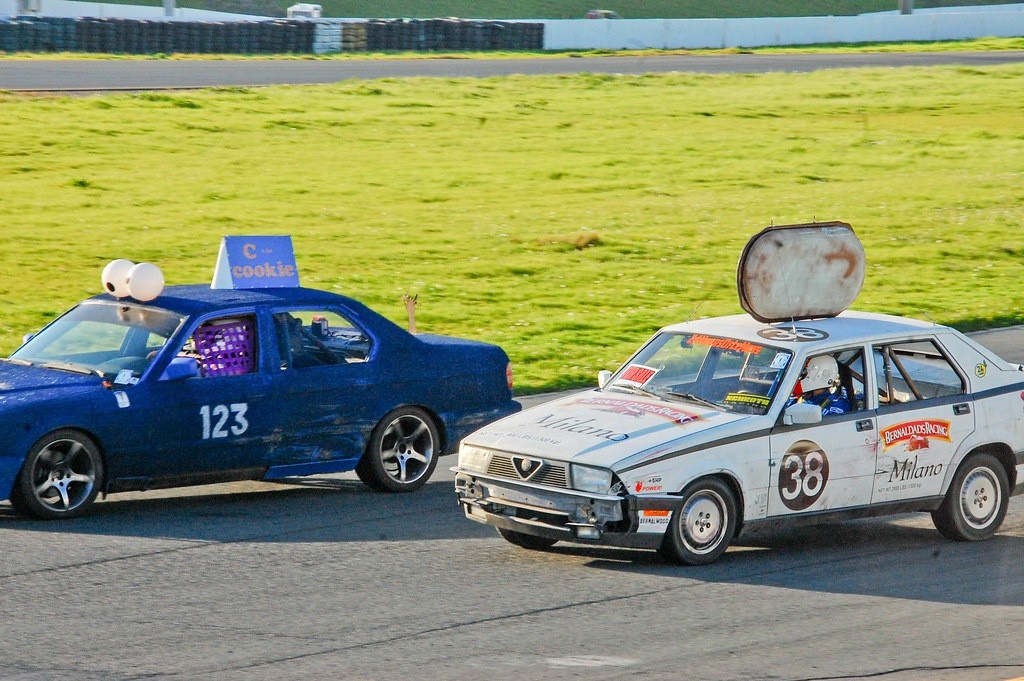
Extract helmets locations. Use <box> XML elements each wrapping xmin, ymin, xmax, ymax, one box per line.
<box><xmin>793</xmin><ymin>354</ymin><xmax>840</xmax><ymax>395</ymax></box>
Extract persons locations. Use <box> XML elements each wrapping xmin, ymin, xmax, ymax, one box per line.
<box><xmin>786</xmin><ymin>355</ymin><xmax>852</xmax><ymax>416</ymax></box>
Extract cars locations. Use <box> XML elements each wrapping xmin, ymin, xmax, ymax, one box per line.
<box><xmin>0</xmin><ymin>233</ymin><xmax>524</xmax><ymax>524</ymax></box>
<box><xmin>447</xmin><ymin>219</ymin><xmax>1024</xmax><ymax>569</ymax></box>
